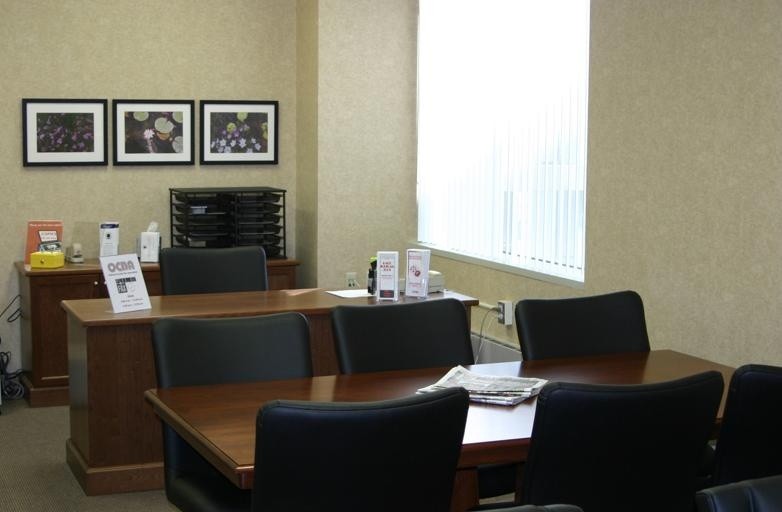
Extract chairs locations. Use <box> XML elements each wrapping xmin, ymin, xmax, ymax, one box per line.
<box><xmin>515</xmin><ymin>290</ymin><xmax>651</xmax><ymax>360</ymax></box>
<box><xmin>160</xmin><ymin>245</ymin><xmax>269</xmax><ymax>296</ymax></box>
<box><xmin>330</xmin><ymin>298</ymin><xmax>479</xmax><ymax>375</ymax></box>
<box><xmin>151</xmin><ymin>311</ymin><xmax>309</xmax><ymax>511</ymax></box>
<box><xmin>527</xmin><ymin>369</ymin><xmax>724</xmax><ymax>512</ymax></box>
<box><xmin>714</xmin><ymin>364</ymin><xmax>782</xmax><ymax>487</ymax></box>
<box><xmin>252</xmin><ymin>387</ymin><xmax>470</xmax><ymax>512</ymax></box>
<box><xmin>690</xmin><ymin>474</ymin><xmax>782</xmax><ymax>512</ymax></box>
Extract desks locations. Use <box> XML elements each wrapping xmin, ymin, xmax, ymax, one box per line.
<box><xmin>62</xmin><ymin>290</ymin><xmax>478</xmax><ymax>498</ymax></box>
<box><xmin>144</xmin><ymin>349</ymin><xmax>737</xmax><ymax>512</ymax></box>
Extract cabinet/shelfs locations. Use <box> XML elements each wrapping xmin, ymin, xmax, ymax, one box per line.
<box><xmin>13</xmin><ymin>260</ymin><xmax>301</xmax><ymax>410</ymax></box>
<box><xmin>167</xmin><ymin>186</ymin><xmax>288</xmax><ymax>260</ymax></box>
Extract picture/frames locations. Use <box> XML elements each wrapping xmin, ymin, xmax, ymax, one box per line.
<box><xmin>200</xmin><ymin>100</ymin><xmax>279</xmax><ymax>165</ymax></box>
<box><xmin>112</xmin><ymin>99</ymin><xmax>195</xmax><ymax>165</ymax></box>
<box><xmin>21</xmin><ymin>97</ymin><xmax>108</xmax><ymax>166</ymax></box>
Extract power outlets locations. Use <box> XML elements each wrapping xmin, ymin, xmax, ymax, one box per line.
<box><xmin>498</xmin><ymin>300</ymin><xmax>513</xmax><ymax>325</ymax></box>
